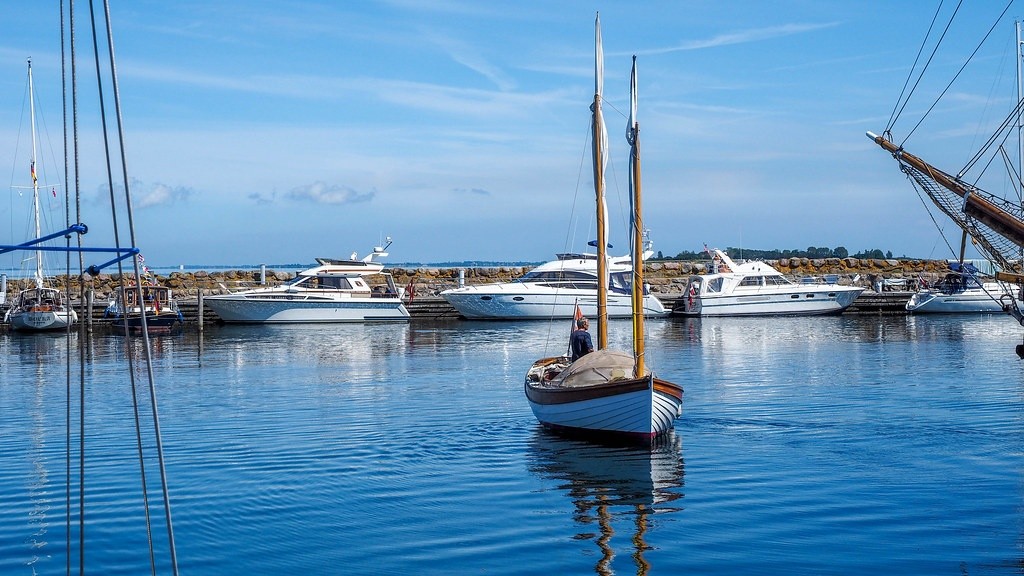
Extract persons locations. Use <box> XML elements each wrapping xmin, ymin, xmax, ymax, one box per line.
<box><xmin>313</xmin><ymin>277</ymin><xmax>319</xmax><ymax>288</ymax></box>
<box><xmin>570</xmin><ymin>315</ymin><xmax>594</xmax><ymax>363</ymax></box>
<box><xmin>687</xmin><ymin>283</ymin><xmax>697</xmax><ymax>312</ymax></box>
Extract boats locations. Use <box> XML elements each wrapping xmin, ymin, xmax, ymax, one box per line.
<box><xmin>906</xmin><ymin>279</ymin><xmax>1024</xmax><ymax>315</ymax></box>
<box><xmin>439</xmin><ymin>255</ymin><xmax>666</xmax><ymax>319</ymax></box>
<box><xmin>202</xmin><ymin>238</ymin><xmax>410</xmax><ymax>325</ymax></box>
<box><xmin>859</xmin><ymin>0</ymin><xmax>1024</xmax><ymax>361</ymax></box>
<box><xmin>107</xmin><ymin>285</ymin><xmax>182</xmax><ymax>331</ymax></box>
<box><xmin>680</xmin><ymin>245</ymin><xmax>865</xmax><ymax>317</ymax></box>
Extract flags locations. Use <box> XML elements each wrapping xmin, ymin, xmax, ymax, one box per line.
<box><xmin>570</xmin><ymin>306</ymin><xmax>582</xmax><ymax>334</ymax></box>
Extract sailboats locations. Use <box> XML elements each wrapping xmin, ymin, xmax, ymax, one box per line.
<box><xmin>523</xmin><ymin>13</ymin><xmax>685</xmax><ymax>443</ymax></box>
<box><xmin>5</xmin><ymin>57</ymin><xmax>78</xmax><ymax>334</ymax></box>
<box><xmin>530</xmin><ymin>426</ymin><xmax>683</xmax><ymax>576</ymax></box>
